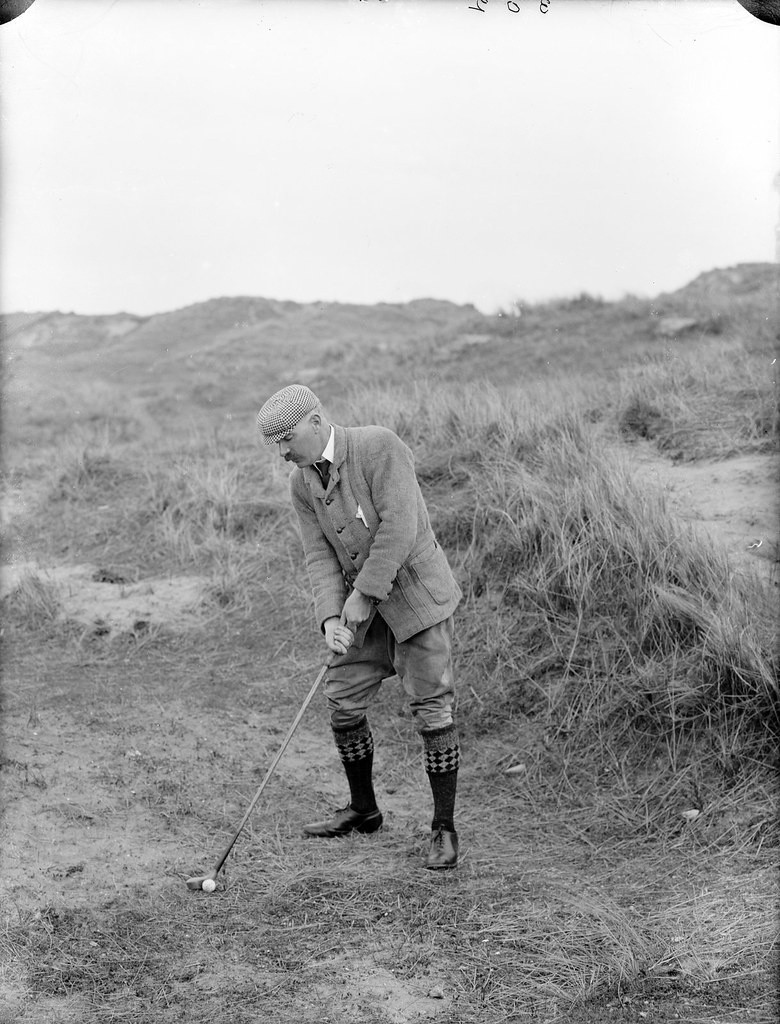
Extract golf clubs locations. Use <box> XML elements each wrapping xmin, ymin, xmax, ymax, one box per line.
<box><xmin>184</xmin><ymin>652</ymin><xmax>338</xmax><ymax>890</ymax></box>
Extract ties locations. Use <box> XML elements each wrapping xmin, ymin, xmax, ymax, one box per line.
<box><xmin>316</xmin><ymin>460</ymin><xmax>331</xmax><ymax>490</ymax></box>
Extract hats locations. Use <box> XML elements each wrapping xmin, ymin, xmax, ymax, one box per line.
<box><xmin>257</xmin><ymin>384</ymin><xmax>319</xmax><ymax>446</ymax></box>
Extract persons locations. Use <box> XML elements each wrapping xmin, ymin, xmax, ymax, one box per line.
<box><xmin>255</xmin><ymin>383</ymin><xmax>464</xmax><ymax>872</ymax></box>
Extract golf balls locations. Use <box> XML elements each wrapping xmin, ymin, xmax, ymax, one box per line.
<box><xmin>201</xmin><ymin>879</ymin><xmax>216</xmax><ymax>893</ymax></box>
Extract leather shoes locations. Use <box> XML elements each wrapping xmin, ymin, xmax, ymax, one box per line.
<box><xmin>303</xmin><ymin>802</ymin><xmax>383</xmax><ymax>837</ymax></box>
<box><xmin>427</xmin><ymin>830</ymin><xmax>459</xmax><ymax>868</ymax></box>
